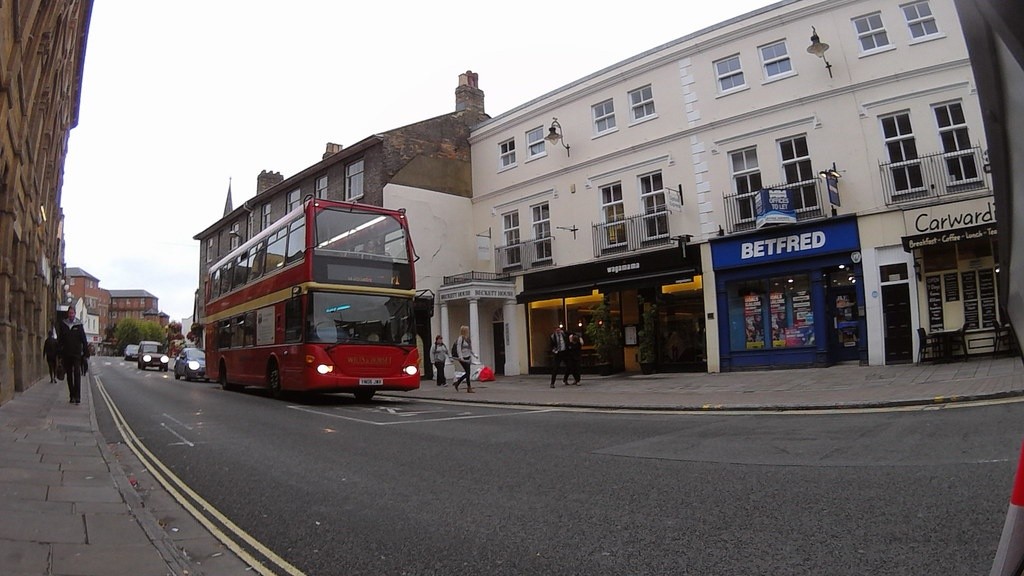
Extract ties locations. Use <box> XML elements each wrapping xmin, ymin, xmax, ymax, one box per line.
<box><xmin>558</xmin><ymin>335</ymin><xmax>561</xmax><ymax>352</ymax></box>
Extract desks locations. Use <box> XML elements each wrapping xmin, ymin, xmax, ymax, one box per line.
<box><xmin>927</xmin><ymin>329</ymin><xmax>961</xmax><ymax>364</ymax></box>
<box><xmin>581</xmin><ymin>346</ymin><xmax>597</xmax><ymax>366</ymax></box>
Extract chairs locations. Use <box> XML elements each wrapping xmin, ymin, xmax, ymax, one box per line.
<box><xmin>916</xmin><ymin>319</ymin><xmax>1014</xmax><ymax>367</ymax></box>
<box><xmin>581</xmin><ymin>354</ymin><xmax>589</xmax><ymax>367</ymax></box>
<box><xmin>377</xmin><ymin>251</ymin><xmax>393</xmax><ymax>262</ymax></box>
<box><xmin>590</xmin><ymin>353</ymin><xmax>601</xmax><ymax>366</ymax></box>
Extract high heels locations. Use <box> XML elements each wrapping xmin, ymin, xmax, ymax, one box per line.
<box><xmin>453</xmin><ymin>382</ymin><xmax>459</xmax><ymax>391</ymax></box>
<box><xmin>467</xmin><ymin>385</ymin><xmax>475</xmax><ymax>393</ymax></box>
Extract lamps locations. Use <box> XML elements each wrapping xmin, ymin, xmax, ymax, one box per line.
<box><xmin>806</xmin><ymin>26</ymin><xmax>832</xmax><ymax>78</ymax></box>
<box><xmin>913</xmin><ymin>261</ymin><xmax>922</xmax><ymax>281</ymax></box>
<box><xmin>544</xmin><ymin>117</ymin><xmax>570</xmax><ymax>157</ymax></box>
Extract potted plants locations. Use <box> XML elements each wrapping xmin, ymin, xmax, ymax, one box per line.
<box><xmin>636</xmin><ymin>294</ymin><xmax>659</xmax><ymax>375</ymax></box>
<box><xmin>584</xmin><ymin>302</ymin><xmax>622</xmax><ymax>376</ymax></box>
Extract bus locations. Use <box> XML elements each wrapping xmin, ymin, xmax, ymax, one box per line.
<box><xmin>204</xmin><ymin>194</ymin><xmax>434</xmax><ymax>402</ymax></box>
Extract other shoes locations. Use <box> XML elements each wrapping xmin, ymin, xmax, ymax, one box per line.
<box><xmin>49</xmin><ymin>379</ymin><xmax>58</xmax><ymax>384</ymax></box>
<box><xmin>69</xmin><ymin>397</ymin><xmax>74</xmax><ymax>403</ymax></box>
<box><xmin>573</xmin><ymin>381</ymin><xmax>580</xmax><ymax>386</ymax></box>
<box><xmin>75</xmin><ymin>400</ymin><xmax>81</xmax><ymax>403</ymax></box>
<box><xmin>443</xmin><ymin>383</ymin><xmax>448</xmax><ymax>386</ymax></box>
<box><xmin>550</xmin><ymin>384</ymin><xmax>555</xmax><ymax>388</ymax></box>
<box><xmin>563</xmin><ymin>380</ymin><xmax>570</xmax><ymax>385</ymax></box>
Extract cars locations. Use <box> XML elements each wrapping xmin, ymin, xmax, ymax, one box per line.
<box><xmin>125</xmin><ymin>345</ymin><xmax>138</xmax><ymax>360</ymax></box>
<box><xmin>138</xmin><ymin>341</ymin><xmax>168</xmax><ymax>372</ymax></box>
<box><xmin>174</xmin><ymin>347</ymin><xmax>210</xmax><ymax>382</ymax></box>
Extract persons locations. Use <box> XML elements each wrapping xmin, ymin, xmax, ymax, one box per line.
<box><xmin>430</xmin><ymin>335</ymin><xmax>450</xmax><ymax>387</ymax></box>
<box><xmin>550</xmin><ymin>326</ymin><xmax>584</xmax><ymax>388</ymax></box>
<box><xmin>452</xmin><ymin>326</ymin><xmax>478</xmax><ymax>393</ymax></box>
<box><xmin>56</xmin><ymin>306</ymin><xmax>90</xmax><ymax>404</ymax></box>
<box><xmin>43</xmin><ymin>331</ymin><xmax>57</xmax><ymax>384</ymax></box>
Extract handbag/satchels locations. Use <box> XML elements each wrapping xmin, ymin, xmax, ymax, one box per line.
<box><xmin>56</xmin><ymin>358</ymin><xmax>66</xmax><ymax>380</ymax></box>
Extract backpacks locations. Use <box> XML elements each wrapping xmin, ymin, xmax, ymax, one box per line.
<box><xmin>451</xmin><ymin>335</ymin><xmax>464</xmax><ymax>358</ymax></box>
